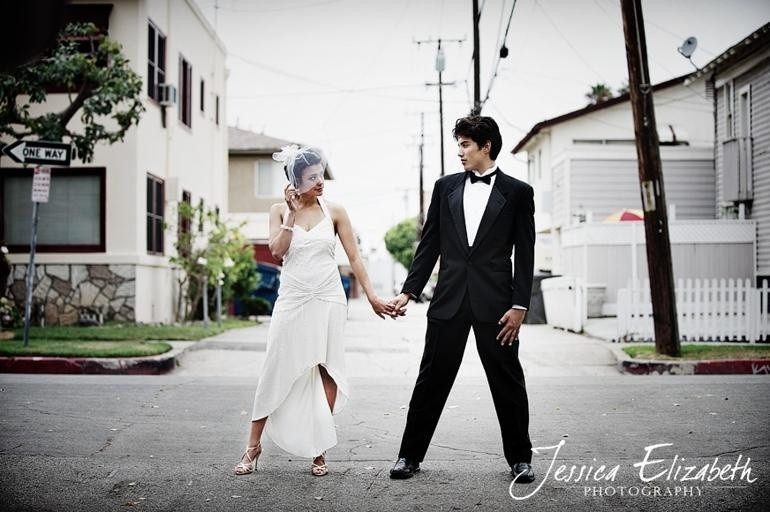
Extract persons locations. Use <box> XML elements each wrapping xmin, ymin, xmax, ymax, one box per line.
<box><xmin>233</xmin><ymin>150</ymin><xmax>401</xmax><ymax>477</ymax></box>
<box><xmin>386</xmin><ymin>115</ymin><xmax>536</xmax><ymax>484</ymax></box>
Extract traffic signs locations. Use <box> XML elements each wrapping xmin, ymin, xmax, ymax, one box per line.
<box><xmin>2</xmin><ymin>140</ymin><xmax>71</xmax><ymax>166</ymax></box>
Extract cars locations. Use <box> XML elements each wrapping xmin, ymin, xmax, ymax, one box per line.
<box><xmin>230</xmin><ymin>261</ymin><xmax>351</xmax><ymax>312</ymax></box>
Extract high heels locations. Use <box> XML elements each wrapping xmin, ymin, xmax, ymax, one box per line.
<box><xmin>234</xmin><ymin>443</ymin><xmax>261</xmax><ymax>475</ymax></box>
<box><xmin>312</xmin><ymin>451</ymin><xmax>328</xmax><ymax>476</ymax></box>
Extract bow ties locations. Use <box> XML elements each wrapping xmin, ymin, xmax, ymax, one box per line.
<box><xmin>470</xmin><ymin>172</ymin><xmax>491</xmax><ymax>185</ymax></box>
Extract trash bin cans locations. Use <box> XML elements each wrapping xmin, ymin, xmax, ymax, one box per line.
<box><xmin>233</xmin><ymin>260</ymin><xmax>282</xmax><ymax>316</ymax></box>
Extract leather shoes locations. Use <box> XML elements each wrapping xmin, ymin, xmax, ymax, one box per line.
<box><xmin>511</xmin><ymin>463</ymin><xmax>535</xmax><ymax>483</ymax></box>
<box><xmin>390</xmin><ymin>458</ymin><xmax>420</xmax><ymax>479</ymax></box>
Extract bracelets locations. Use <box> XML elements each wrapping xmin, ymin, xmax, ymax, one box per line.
<box><xmin>280</xmin><ymin>225</ymin><xmax>294</xmax><ymax>232</ymax></box>
